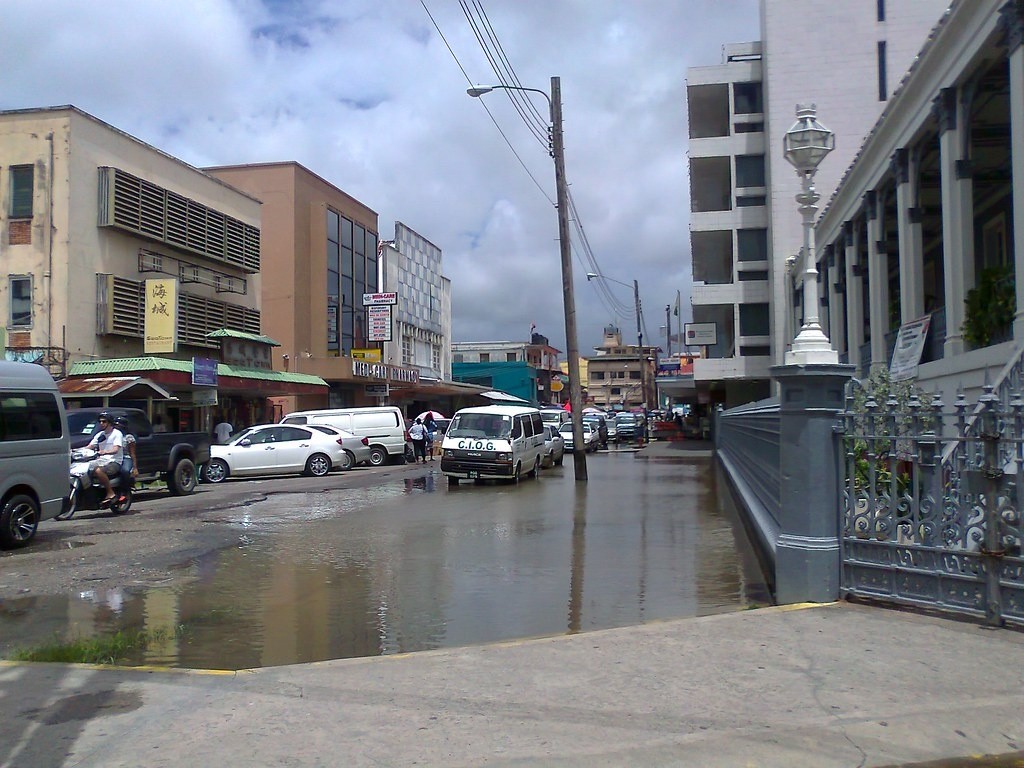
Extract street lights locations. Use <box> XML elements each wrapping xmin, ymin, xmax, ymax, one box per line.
<box><xmin>589</xmin><ymin>275</ymin><xmax>650</xmax><ymax>443</ymax></box>
<box><xmin>467</xmin><ymin>74</ymin><xmax>592</xmax><ymax>480</ymax></box>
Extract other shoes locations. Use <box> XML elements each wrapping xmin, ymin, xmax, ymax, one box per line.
<box><xmin>118</xmin><ymin>495</ymin><xmax>126</xmax><ymax>501</ymax></box>
<box><xmin>416</xmin><ymin>462</ymin><xmax>419</xmax><ymax>464</ymax></box>
<box><xmin>422</xmin><ymin>460</ymin><xmax>428</xmax><ymax>464</ymax></box>
<box><xmin>430</xmin><ymin>459</ymin><xmax>435</xmax><ymax>461</ymax></box>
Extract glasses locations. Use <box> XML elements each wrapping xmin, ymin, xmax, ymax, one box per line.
<box><xmin>98</xmin><ymin>419</ymin><xmax>108</xmax><ymax>423</ymax></box>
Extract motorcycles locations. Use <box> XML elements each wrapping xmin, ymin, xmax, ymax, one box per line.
<box><xmin>56</xmin><ymin>433</ymin><xmax>133</xmax><ymax>521</ymax></box>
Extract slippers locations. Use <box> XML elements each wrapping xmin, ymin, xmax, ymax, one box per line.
<box><xmin>100</xmin><ymin>493</ymin><xmax>116</xmax><ymax>505</ymax></box>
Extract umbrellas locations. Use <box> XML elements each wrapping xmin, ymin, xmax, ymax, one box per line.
<box><xmin>415</xmin><ymin>410</ymin><xmax>444</xmax><ymax>420</ymax></box>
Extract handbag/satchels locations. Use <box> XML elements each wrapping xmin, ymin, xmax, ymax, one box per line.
<box><xmin>421</xmin><ymin>424</ymin><xmax>430</xmax><ymax>442</ymax></box>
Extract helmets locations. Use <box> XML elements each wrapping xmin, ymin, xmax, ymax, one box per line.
<box><xmin>98</xmin><ymin>411</ymin><xmax>113</xmax><ymax>423</ymax></box>
<box><xmin>112</xmin><ymin>416</ymin><xmax>129</xmax><ymax>428</ymax></box>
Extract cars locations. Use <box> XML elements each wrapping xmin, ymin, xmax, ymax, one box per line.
<box><xmin>538</xmin><ymin>409</ymin><xmax>642</xmax><ymax>453</ymax></box>
<box><xmin>305</xmin><ymin>424</ymin><xmax>371</xmax><ymax>469</ymax></box>
<box><xmin>200</xmin><ymin>424</ymin><xmax>346</xmax><ymax>484</ymax></box>
<box><xmin>544</xmin><ymin>423</ymin><xmax>566</xmax><ymax>468</ymax></box>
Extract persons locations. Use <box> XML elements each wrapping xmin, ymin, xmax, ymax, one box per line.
<box><xmin>213</xmin><ymin>416</ymin><xmax>233</xmax><ymax>443</ymax></box>
<box><xmin>72</xmin><ymin>411</ymin><xmax>139</xmax><ymax>504</ymax></box>
<box><xmin>423</xmin><ymin>412</ymin><xmax>439</xmax><ymax>461</ymax></box>
<box><xmin>407</xmin><ymin>418</ymin><xmax>428</xmax><ymax>465</ymax></box>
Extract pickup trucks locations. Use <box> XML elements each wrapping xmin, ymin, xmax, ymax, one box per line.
<box><xmin>66</xmin><ymin>408</ymin><xmax>211</xmax><ymax>497</ymax></box>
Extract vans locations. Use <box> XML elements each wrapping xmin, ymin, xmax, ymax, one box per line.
<box><xmin>441</xmin><ymin>406</ymin><xmax>547</xmax><ymax>484</ymax></box>
<box><xmin>279</xmin><ymin>407</ymin><xmax>409</xmax><ymax>466</ymax></box>
<box><xmin>0</xmin><ymin>361</ymin><xmax>69</xmax><ymax>549</ymax></box>
